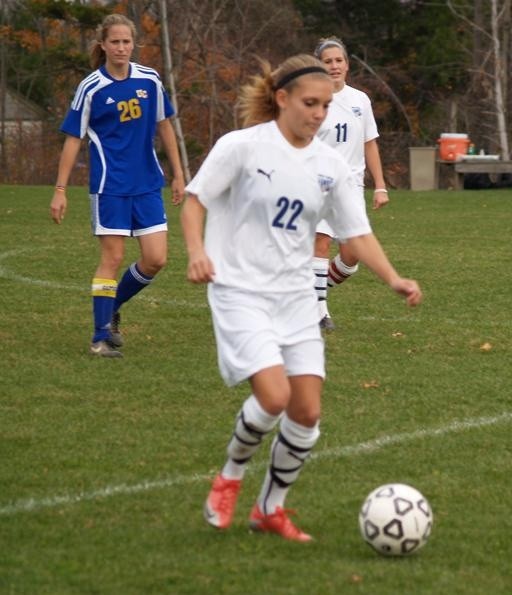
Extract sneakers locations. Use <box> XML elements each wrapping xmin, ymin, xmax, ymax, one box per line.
<box><xmin>203</xmin><ymin>470</ymin><xmax>240</xmax><ymax>528</ymax></box>
<box><xmin>110</xmin><ymin>312</ymin><xmax>123</xmax><ymax>347</ymax></box>
<box><xmin>249</xmin><ymin>505</ymin><xmax>312</xmax><ymax>541</ymax></box>
<box><xmin>90</xmin><ymin>341</ymin><xmax>123</xmax><ymax>358</ymax></box>
<box><xmin>318</xmin><ymin>299</ymin><xmax>332</xmax><ymax>326</ymax></box>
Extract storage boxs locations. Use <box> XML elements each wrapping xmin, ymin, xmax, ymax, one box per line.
<box><xmin>436</xmin><ymin>133</ymin><xmax>471</xmax><ymax>161</ymax></box>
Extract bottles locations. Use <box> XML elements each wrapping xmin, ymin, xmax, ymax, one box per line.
<box><xmin>468</xmin><ymin>143</ymin><xmax>475</xmax><ymax>155</ymax></box>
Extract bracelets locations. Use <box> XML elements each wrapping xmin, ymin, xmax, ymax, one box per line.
<box><xmin>54</xmin><ymin>185</ymin><xmax>66</xmax><ymax>193</ymax></box>
<box><xmin>374</xmin><ymin>188</ymin><xmax>389</xmax><ymax>193</ymax></box>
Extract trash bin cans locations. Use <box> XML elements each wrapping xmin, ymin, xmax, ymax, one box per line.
<box><xmin>408</xmin><ymin>147</ymin><xmax>439</xmax><ymax>192</ymax></box>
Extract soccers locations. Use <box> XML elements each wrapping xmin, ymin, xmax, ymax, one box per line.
<box><xmin>359</xmin><ymin>484</ymin><xmax>433</xmax><ymax>557</ymax></box>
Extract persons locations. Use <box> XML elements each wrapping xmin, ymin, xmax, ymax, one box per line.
<box><xmin>49</xmin><ymin>13</ymin><xmax>187</xmax><ymax>360</ymax></box>
<box><xmin>311</xmin><ymin>33</ymin><xmax>392</xmax><ymax>332</ymax></box>
<box><xmin>177</xmin><ymin>52</ymin><xmax>423</xmax><ymax>543</ymax></box>
